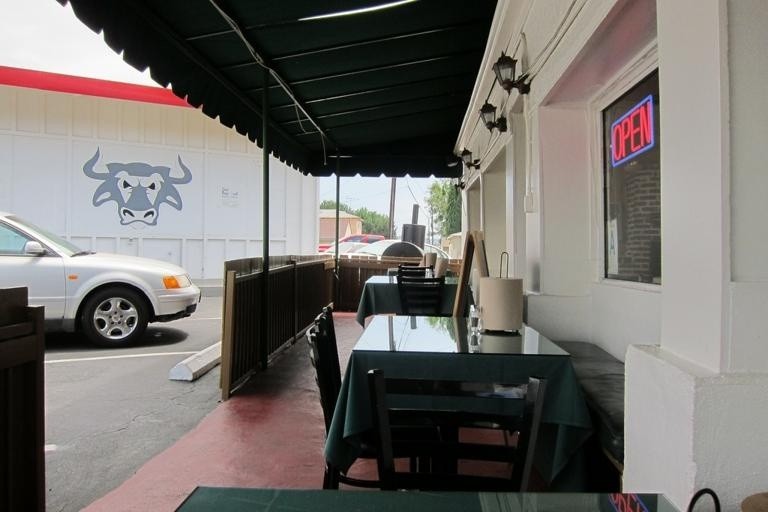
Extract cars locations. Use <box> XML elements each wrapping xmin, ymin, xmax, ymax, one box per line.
<box><xmin>0</xmin><ymin>210</ymin><xmax>202</xmax><ymax>350</ymax></box>
<box><xmin>318</xmin><ymin>231</ymin><xmax>462</xmax><ymax>259</ymax></box>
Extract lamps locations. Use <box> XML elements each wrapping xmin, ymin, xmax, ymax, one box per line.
<box><xmin>490</xmin><ymin>47</ymin><xmax>534</xmax><ymax>96</ymax></box>
<box><xmin>461</xmin><ymin>146</ymin><xmax>480</xmax><ymax>170</ymax></box>
<box><xmin>480</xmin><ymin>101</ymin><xmax>509</xmax><ymax>134</ymax></box>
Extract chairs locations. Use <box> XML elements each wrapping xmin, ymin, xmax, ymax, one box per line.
<box><xmin>396</xmin><ymin>262</ymin><xmax>446</xmax><ymax>315</ymax></box>
<box><xmin>364</xmin><ymin>367</ymin><xmax>545</xmax><ymax>494</ymax></box>
<box><xmin>305</xmin><ymin>304</ymin><xmax>459</xmax><ymax>494</ymax></box>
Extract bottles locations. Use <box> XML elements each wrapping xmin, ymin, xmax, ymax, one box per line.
<box><xmin>469</xmin><ymin>305</ymin><xmax>480</xmax><ymax>332</ymax></box>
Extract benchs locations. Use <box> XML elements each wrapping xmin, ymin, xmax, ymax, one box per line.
<box><xmin>557</xmin><ymin>334</ymin><xmax>643</xmax><ymax>474</ymax></box>
